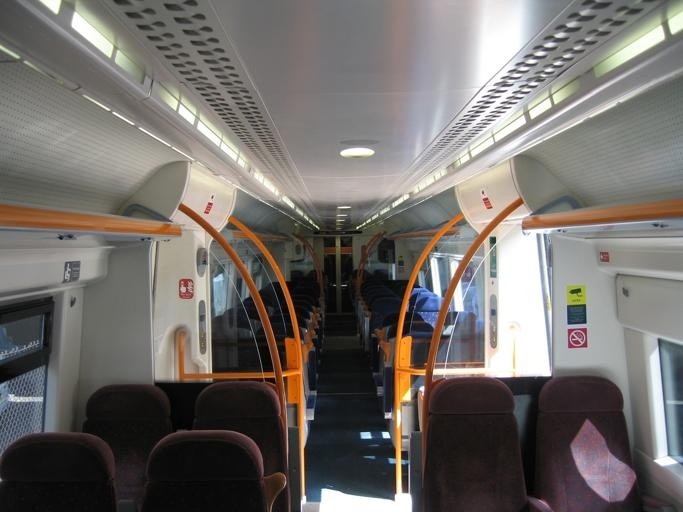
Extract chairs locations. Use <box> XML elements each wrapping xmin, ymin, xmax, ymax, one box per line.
<box><xmin>0</xmin><ymin>269</ymin><xmax>327</xmax><ymax>512</ymax></box>
<box><xmin>351</xmin><ymin>266</ymin><xmax>678</xmax><ymax>511</ymax></box>
